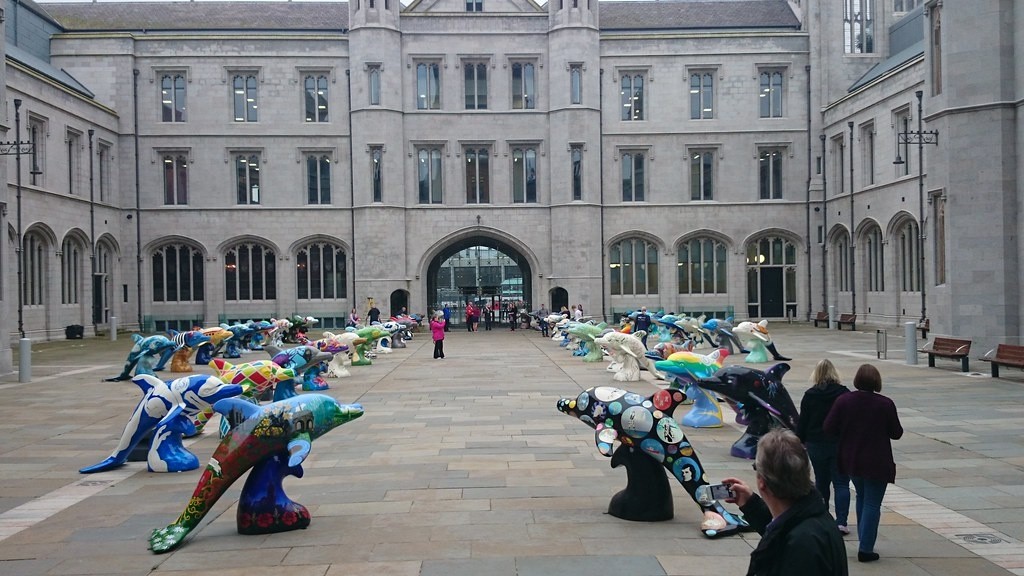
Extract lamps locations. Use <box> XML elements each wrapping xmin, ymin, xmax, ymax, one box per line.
<box><xmin>0</xmin><ymin>141</ymin><xmax>43</xmax><ymax>175</ymax></box>
<box><xmin>892</xmin><ymin>129</ymin><xmax>939</xmax><ymax>165</ymax></box>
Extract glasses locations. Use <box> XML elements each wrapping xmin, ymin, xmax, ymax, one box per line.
<box><xmin>752</xmin><ymin>462</ymin><xmax>768</xmax><ymax>482</ymax></box>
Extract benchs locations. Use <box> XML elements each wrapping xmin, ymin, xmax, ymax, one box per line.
<box><xmin>810</xmin><ymin>312</ymin><xmax>829</xmax><ymax>329</ymax></box>
<box><xmin>916</xmin><ymin>337</ymin><xmax>972</xmax><ymax>372</ymax></box>
<box><xmin>832</xmin><ymin>314</ymin><xmax>857</xmax><ymax>331</ymax></box>
<box><xmin>977</xmin><ymin>344</ymin><xmax>1024</xmax><ymax>378</ymax></box>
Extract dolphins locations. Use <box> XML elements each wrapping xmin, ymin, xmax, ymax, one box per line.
<box><xmin>540</xmin><ymin>304</ymin><xmax>792</xmax><ymax>414</ymax></box>
<box><xmin>699</xmin><ymin>362</ymin><xmax>802</xmax><ymax>459</ymax></box>
<box><xmin>78</xmin><ymin>372</ymin><xmax>243</xmax><ymax>475</ymax></box>
<box><xmin>557</xmin><ymin>384</ymin><xmax>754</xmax><ymax>540</ymax></box>
<box><xmin>147</xmin><ymin>391</ymin><xmax>366</xmax><ymax>554</ymax></box>
<box><xmin>101</xmin><ymin>312</ymin><xmax>320</xmax><ymax>382</ymax></box>
<box><xmin>181</xmin><ymin>314</ymin><xmax>423</xmax><ymax>441</ymax></box>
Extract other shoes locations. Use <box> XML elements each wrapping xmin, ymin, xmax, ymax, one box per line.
<box><xmin>443</xmin><ymin>356</ymin><xmax>446</xmax><ymax>359</ymax></box>
<box><xmin>838</xmin><ymin>524</ymin><xmax>849</xmax><ymax>534</ymax></box>
<box><xmin>437</xmin><ymin>357</ymin><xmax>441</xmax><ymax>359</ymax></box>
<box><xmin>858</xmin><ymin>552</ymin><xmax>879</xmax><ymax>562</ymax></box>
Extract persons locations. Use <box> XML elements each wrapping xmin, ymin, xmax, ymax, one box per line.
<box><xmin>346</xmin><ymin>309</ymin><xmax>359</xmax><ymax>329</ymax></box>
<box><xmin>395</xmin><ymin>306</ymin><xmax>408</xmax><ymax>318</ymax></box>
<box><xmin>634</xmin><ymin>307</ymin><xmax>651</xmax><ymax>351</ymax></box>
<box><xmin>560</xmin><ymin>304</ymin><xmax>583</xmax><ymax>321</ymax></box>
<box><xmin>620</xmin><ymin>314</ymin><xmax>631</xmax><ymax>334</ymax></box>
<box><xmin>538</xmin><ymin>304</ymin><xmax>550</xmax><ymax>337</ymax></box>
<box><xmin>824</xmin><ymin>364</ymin><xmax>904</xmax><ymax>562</ymax></box>
<box><xmin>722</xmin><ymin>429</ymin><xmax>849</xmax><ymax>576</ymax></box>
<box><xmin>485</xmin><ymin>305</ymin><xmax>492</xmax><ymax>331</ymax></box>
<box><xmin>430</xmin><ymin>317</ymin><xmax>446</xmax><ymax>359</ymax></box>
<box><xmin>366</xmin><ymin>302</ymin><xmax>381</xmax><ymax>326</ymax></box>
<box><xmin>442</xmin><ymin>304</ymin><xmax>451</xmax><ymax>332</ymax></box>
<box><xmin>507</xmin><ymin>302</ymin><xmax>518</xmax><ymax>332</ymax></box>
<box><xmin>466</xmin><ymin>301</ymin><xmax>480</xmax><ymax>332</ymax></box>
<box><xmin>797</xmin><ymin>360</ymin><xmax>852</xmax><ymax>535</ymax></box>
<box><xmin>296</xmin><ymin>328</ymin><xmax>309</xmax><ymax>344</ymax></box>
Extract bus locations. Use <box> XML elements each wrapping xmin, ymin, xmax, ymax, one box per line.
<box><xmin>475</xmin><ymin>297</ymin><xmax>523</xmax><ymax>317</ymax></box>
<box><xmin>436</xmin><ymin>294</ymin><xmax>468</xmax><ymax>309</ymax></box>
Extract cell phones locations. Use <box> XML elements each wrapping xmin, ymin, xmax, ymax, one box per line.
<box><xmin>707</xmin><ymin>483</ymin><xmax>737</xmax><ymax>502</ymax></box>
